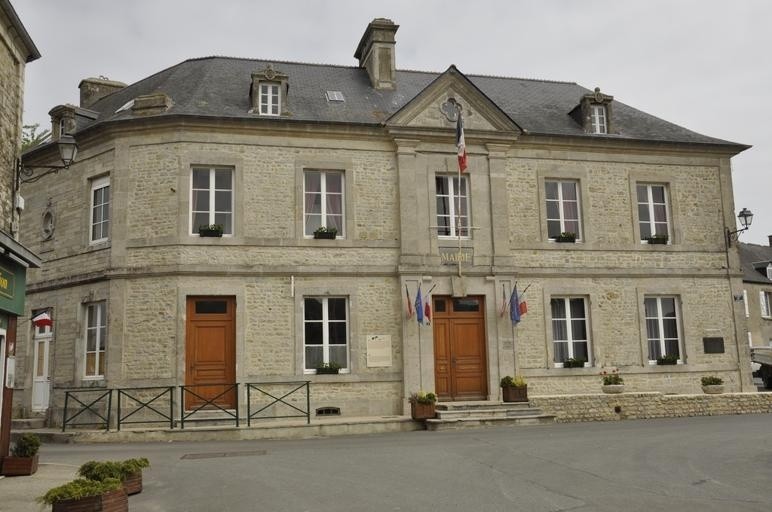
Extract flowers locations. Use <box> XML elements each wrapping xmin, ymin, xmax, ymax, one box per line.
<box><xmin>600</xmin><ymin>367</ymin><xmax>624</xmax><ymax>384</ymax></box>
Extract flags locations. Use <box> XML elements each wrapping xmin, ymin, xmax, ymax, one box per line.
<box><xmin>406</xmin><ymin>288</ymin><xmax>413</xmax><ymax>315</ymax></box>
<box><xmin>454</xmin><ymin>109</ymin><xmax>468</xmax><ymax>172</ymax></box>
<box><xmin>509</xmin><ymin>284</ymin><xmax>520</xmax><ymax>324</ymax></box>
<box><xmin>500</xmin><ymin>288</ymin><xmax>506</xmax><ymax>318</ymax></box>
<box><xmin>424</xmin><ymin>290</ymin><xmax>432</xmax><ymax>326</ymax></box>
<box><xmin>518</xmin><ymin>292</ymin><xmax>528</xmax><ymax>316</ymax></box>
<box><xmin>415</xmin><ymin>287</ymin><xmax>424</xmax><ymax>326</ymax></box>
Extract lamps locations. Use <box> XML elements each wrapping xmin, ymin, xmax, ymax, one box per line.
<box><xmin>727</xmin><ymin>205</ymin><xmax>753</xmax><ymax>249</ymax></box>
<box><xmin>13</xmin><ymin>134</ymin><xmax>78</xmax><ymax>192</ymax></box>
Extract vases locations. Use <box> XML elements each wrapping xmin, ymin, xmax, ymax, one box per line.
<box><xmin>600</xmin><ymin>385</ymin><xmax>624</xmax><ymax>393</ymax></box>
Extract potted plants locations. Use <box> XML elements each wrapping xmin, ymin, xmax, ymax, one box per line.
<box><xmin>199</xmin><ymin>222</ymin><xmax>224</xmax><ymax>236</ymax></box>
<box><xmin>702</xmin><ymin>375</ymin><xmax>724</xmax><ymax>393</ymax></box>
<box><xmin>657</xmin><ymin>355</ymin><xmax>679</xmax><ymax>365</ymax></box>
<box><xmin>564</xmin><ymin>356</ymin><xmax>585</xmax><ymax>368</ymax></box>
<box><xmin>313</xmin><ymin>227</ymin><xmax>338</xmax><ymax>239</ymax></box>
<box><xmin>646</xmin><ymin>233</ymin><xmax>668</xmax><ymax>244</ymax></box>
<box><xmin>409</xmin><ymin>389</ymin><xmax>436</xmax><ymax>418</ymax></box>
<box><xmin>501</xmin><ymin>374</ymin><xmax>528</xmax><ymax>402</ymax></box>
<box><xmin>554</xmin><ymin>231</ymin><xmax>575</xmax><ymax>242</ymax></box>
<box><xmin>317</xmin><ymin>362</ymin><xmax>341</xmax><ymax>375</ymax></box>
<box><xmin>4</xmin><ymin>432</ymin><xmax>149</xmax><ymax>512</ymax></box>
